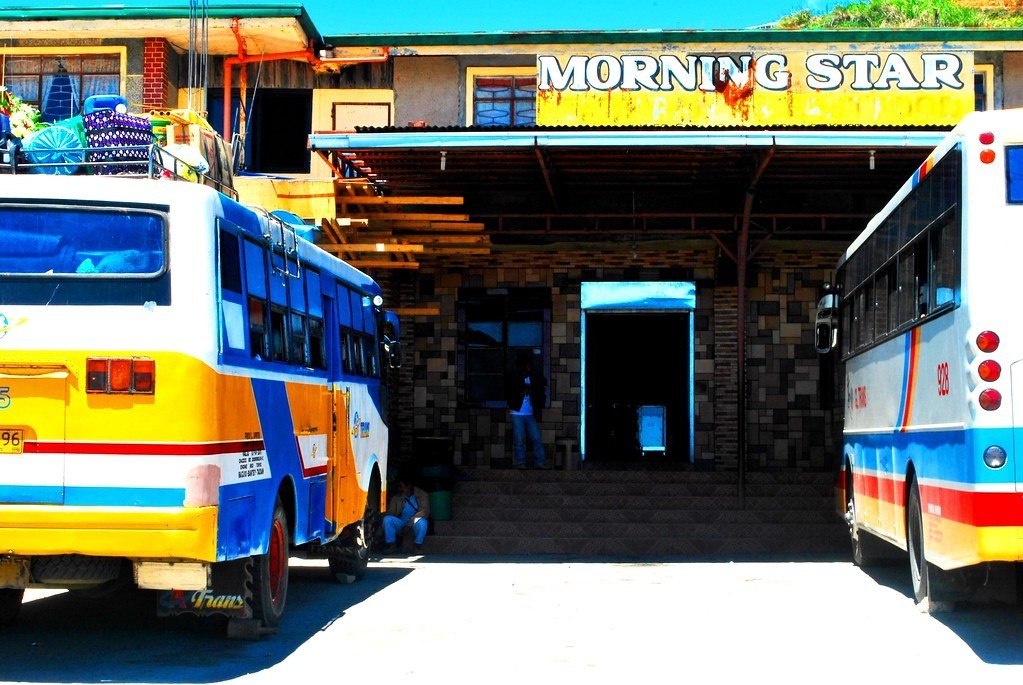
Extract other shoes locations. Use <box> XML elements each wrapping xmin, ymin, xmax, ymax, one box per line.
<box><xmin>517</xmin><ymin>464</ymin><xmax>529</xmax><ymax>470</ymax></box>
<box><xmin>535</xmin><ymin>465</ymin><xmax>552</xmax><ymax>470</ymax></box>
<box><xmin>415</xmin><ymin>544</ymin><xmax>425</xmax><ymax>555</ymax></box>
<box><xmin>383</xmin><ymin>543</ymin><xmax>398</xmax><ymax>555</ymax></box>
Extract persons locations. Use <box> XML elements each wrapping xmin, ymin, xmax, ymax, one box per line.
<box><xmin>382</xmin><ymin>476</ymin><xmax>430</xmax><ymax>555</ymax></box>
<box><xmin>507</xmin><ymin>359</ymin><xmax>550</xmax><ymax>469</ymax></box>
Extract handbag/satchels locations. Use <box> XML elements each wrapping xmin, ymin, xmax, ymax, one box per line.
<box><xmin>426</xmin><ymin>516</ymin><xmax>436</xmax><ymax>536</ymax></box>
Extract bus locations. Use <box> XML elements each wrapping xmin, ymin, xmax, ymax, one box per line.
<box><xmin>812</xmin><ymin>108</ymin><xmax>1022</xmax><ymax>612</ymax></box>
<box><xmin>0</xmin><ymin>143</ymin><xmax>404</xmax><ymax>637</ymax></box>
